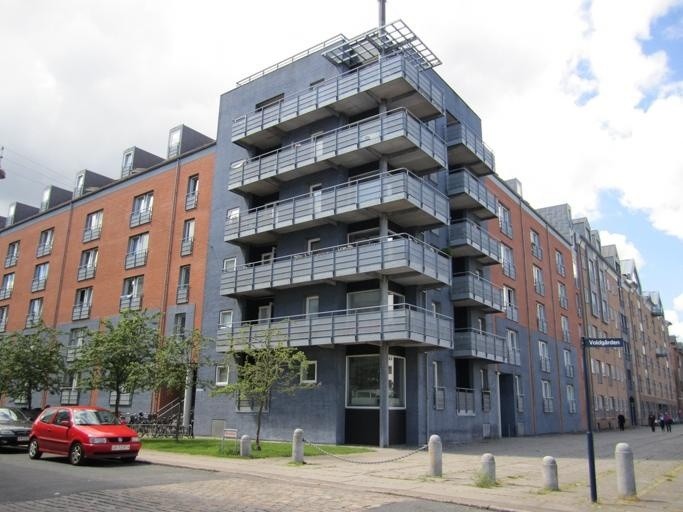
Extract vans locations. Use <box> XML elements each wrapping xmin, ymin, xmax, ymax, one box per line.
<box><xmin>349</xmin><ymin>387</ymin><xmax>402</xmax><ymax>408</ymax></box>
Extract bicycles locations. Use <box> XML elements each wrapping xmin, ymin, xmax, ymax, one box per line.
<box><xmin>115</xmin><ymin>409</ymin><xmax>193</xmax><ymax>439</ymax></box>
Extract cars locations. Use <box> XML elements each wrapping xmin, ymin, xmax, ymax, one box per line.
<box><xmin>1</xmin><ymin>407</ymin><xmax>37</xmax><ymax>455</ymax></box>
<box><xmin>27</xmin><ymin>404</ymin><xmax>143</xmax><ymax>467</ymax></box>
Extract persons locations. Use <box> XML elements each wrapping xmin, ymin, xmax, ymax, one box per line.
<box><xmin>648</xmin><ymin>410</ymin><xmax>673</xmax><ymax>432</ymax></box>
<box><xmin>617</xmin><ymin>413</ymin><xmax>626</xmax><ymax>431</ymax></box>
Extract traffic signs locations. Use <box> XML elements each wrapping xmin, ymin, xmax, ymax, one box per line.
<box><xmin>582</xmin><ymin>337</ymin><xmax>621</xmax><ymax>347</ymax></box>
